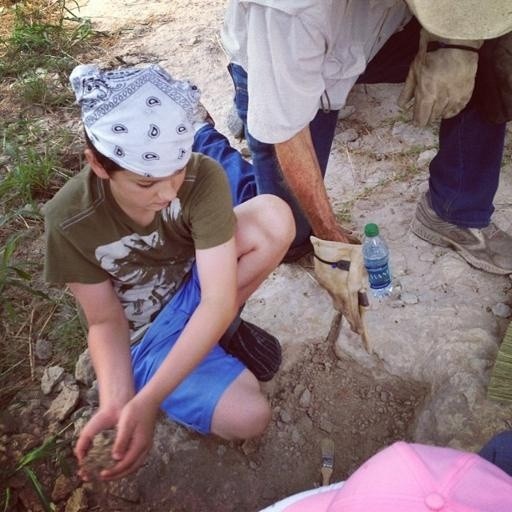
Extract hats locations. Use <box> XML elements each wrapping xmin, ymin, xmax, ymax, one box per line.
<box><xmin>258</xmin><ymin>441</ymin><xmax>511</xmax><ymax>512</ymax></box>
<box><xmin>409</xmin><ymin>1</ymin><xmax>511</xmax><ymax>39</ymax></box>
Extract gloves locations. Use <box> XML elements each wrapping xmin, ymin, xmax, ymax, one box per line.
<box><xmin>396</xmin><ymin>40</ymin><xmax>478</xmax><ymax>128</ymax></box>
<box><xmin>310</xmin><ymin>228</ymin><xmax>370</xmax><ymax>335</ymax></box>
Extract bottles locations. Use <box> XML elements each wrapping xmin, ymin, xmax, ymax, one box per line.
<box><xmin>361</xmin><ymin>223</ymin><xmax>393</xmax><ymax>301</ymax></box>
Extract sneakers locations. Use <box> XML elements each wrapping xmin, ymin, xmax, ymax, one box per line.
<box><xmin>411</xmin><ymin>191</ymin><xmax>511</xmax><ymax>274</ymax></box>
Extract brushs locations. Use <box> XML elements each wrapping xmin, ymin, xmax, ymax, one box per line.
<box><xmin>318</xmin><ymin>439</ymin><xmax>334</xmax><ymax>485</ymax></box>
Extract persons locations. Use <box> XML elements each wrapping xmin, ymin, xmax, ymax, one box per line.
<box><xmin>247</xmin><ymin>429</ymin><xmax>512</xmax><ymax>511</ymax></box>
<box><xmin>40</xmin><ymin>58</ymin><xmax>301</xmax><ymax>485</ymax></box>
<box><xmin>184</xmin><ymin>0</ymin><xmax>512</xmax><ymax>357</ymax></box>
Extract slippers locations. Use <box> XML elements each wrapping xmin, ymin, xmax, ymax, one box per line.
<box><xmin>218</xmin><ymin>305</ymin><xmax>281</xmax><ymax>381</ymax></box>
